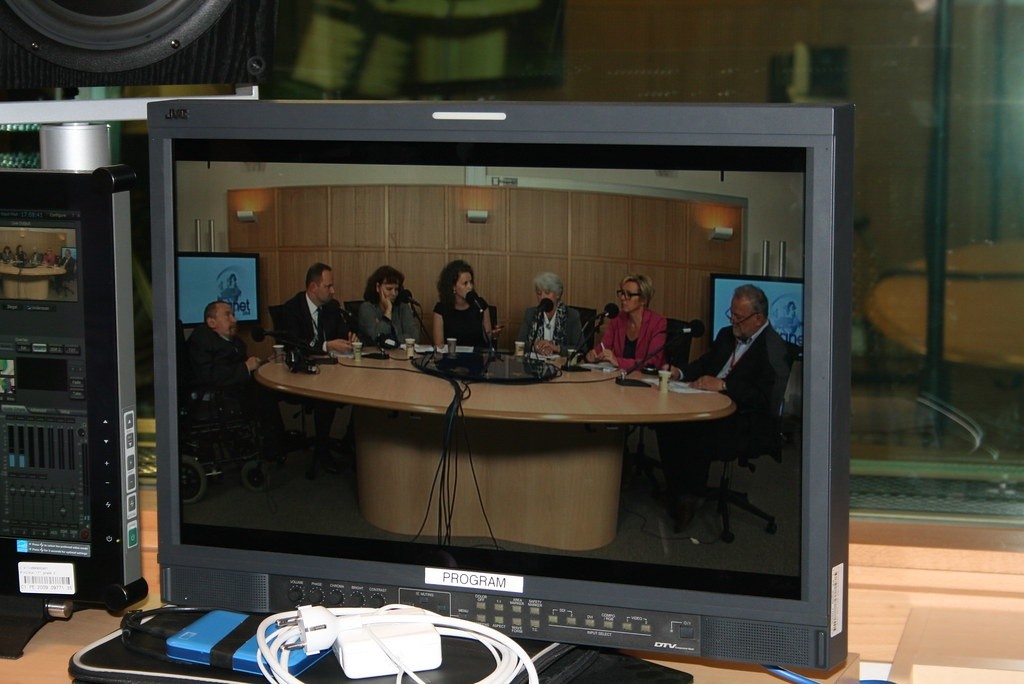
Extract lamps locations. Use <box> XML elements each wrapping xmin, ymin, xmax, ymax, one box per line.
<box><xmin>236</xmin><ymin>211</ymin><xmax>255</xmax><ymax>222</ymax></box>
<box><xmin>468</xmin><ymin>210</ymin><xmax>489</xmax><ymax>222</ymax></box>
<box><xmin>711</xmin><ymin>227</ymin><xmax>734</xmax><ymax>239</ymax></box>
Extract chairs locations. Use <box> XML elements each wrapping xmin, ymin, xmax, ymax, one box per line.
<box><xmin>573</xmin><ymin>306</ymin><xmax>597</xmax><ymax>353</ymax></box>
<box><xmin>675</xmin><ymin>342</ymin><xmax>799</xmax><ymax>542</ymax></box>
<box><xmin>626</xmin><ymin>317</ymin><xmax>692</xmax><ymax>477</ymax></box>
<box><xmin>268</xmin><ymin>304</ymin><xmax>287</xmax><ymax>345</ymax></box>
<box><xmin>54</xmin><ymin>260</ymin><xmax>75</xmax><ymax>295</ymax></box>
<box><xmin>343</xmin><ymin>300</ymin><xmax>365</xmax><ymax>326</ymax></box>
<box><xmin>489</xmin><ymin>305</ymin><xmax>498</xmax><ymax>348</ymax></box>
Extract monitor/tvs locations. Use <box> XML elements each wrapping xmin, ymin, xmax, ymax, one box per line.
<box><xmin>710</xmin><ymin>272</ymin><xmax>806</xmax><ymax>358</ymax></box>
<box><xmin>176</xmin><ymin>251</ymin><xmax>260</xmax><ymax>328</ymax></box>
<box><xmin>60</xmin><ymin>247</ymin><xmax>77</xmax><ymax>260</ymax></box>
<box><xmin>144</xmin><ymin>98</ymin><xmax>857</xmax><ymax>671</ymax></box>
<box><xmin>0</xmin><ymin>164</ymin><xmax>150</xmax><ymax>658</ymax></box>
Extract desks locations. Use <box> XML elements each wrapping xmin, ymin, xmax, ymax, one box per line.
<box><xmin>0</xmin><ymin>259</ymin><xmax>67</xmax><ymax>301</ymax></box>
<box><xmin>254</xmin><ymin>347</ymin><xmax>737</xmax><ymax>552</ymax></box>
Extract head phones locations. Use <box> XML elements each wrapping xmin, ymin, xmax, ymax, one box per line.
<box><xmin>284</xmin><ymin>348</ymin><xmax>320</xmax><ymax>375</ymax></box>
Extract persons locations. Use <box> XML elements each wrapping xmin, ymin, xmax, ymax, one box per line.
<box><xmin>432</xmin><ymin>259</ymin><xmax>493</xmax><ymax>350</ymax></box>
<box><xmin>185</xmin><ymin>300</ymin><xmax>288</xmax><ymax>461</ymax></box>
<box><xmin>0</xmin><ymin>245</ymin><xmax>74</xmax><ymax>293</ymax></box>
<box><xmin>787</xmin><ymin>301</ymin><xmax>797</xmax><ymax>322</ymax></box>
<box><xmin>662</xmin><ymin>283</ymin><xmax>792</xmax><ymax>533</ymax></box>
<box><xmin>584</xmin><ymin>272</ymin><xmax>668</xmax><ymax>370</ymax></box>
<box><xmin>283</xmin><ymin>260</ymin><xmax>361</xmax><ymax>462</ymax></box>
<box><xmin>516</xmin><ymin>271</ymin><xmax>581</xmax><ymax>358</ymax></box>
<box><xmin>358</xmin><ymin>264</ymin><xmax>419</xmax><ymax>344</ymax></box>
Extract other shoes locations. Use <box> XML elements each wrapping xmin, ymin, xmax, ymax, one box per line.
<box><xmin>668</xmin><ymin>493</ymin><xmax>706</xmax><ymax>532</ymax></box>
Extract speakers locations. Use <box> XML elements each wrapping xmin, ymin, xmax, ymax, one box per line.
<box><xmin>0</xmin><ymin>0</ymin><xmax>279</xmax><ymax>92</ymax></box>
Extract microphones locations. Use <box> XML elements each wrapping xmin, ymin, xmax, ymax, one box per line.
<box><xmin>325</xmin><ymin>298</ymin><xmax>353</xmax><ymax>318</ymax></box>
<box><xmin>591</xmin><ymin>302</ymin><xmax>619</xmax><ymax>321</ymax></box>
<box><xmin>249</xmin><ymin>325</ymin><xmax>287</xmax><ymax>343</ymax></box>
<box><xmin>397</xmin><ymin>288</ymin><xmax>421</xmax><ymax>307</ymax></box>
<box><xmin>535</xmin><ymin>298</ymin><xmax>554</xmax><ymax>313</ymax></box>
<box><xmin>662</xmin><ymin>319</ymin><xmax>705</xmax><ymax>338</ymax></box>
<box><xmin>465</xmin><ymin>291</ymin><xmax>483</xmax><ymax>313</ymax></box>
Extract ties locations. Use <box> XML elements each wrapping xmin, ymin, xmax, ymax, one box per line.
<box><xmin>317</xmin><ymin>307</ymin><xmax>324</xmax><ymax>343</ymax></box>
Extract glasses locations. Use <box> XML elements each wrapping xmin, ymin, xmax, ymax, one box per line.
<box><xmin>616</xmin><ymin>289</ymin><xmax>642</xmax><ymax>300</ymax></box>
<box><xmin>724</xmin><ymin>307</ymin><xmax>761</xmax><ymax>326</ymax></box>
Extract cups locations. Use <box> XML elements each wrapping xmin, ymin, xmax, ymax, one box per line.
<box><xmin>447</xmin><ymin>338</ymin><xmax>456</xmax><ymax>353</ymax></box>
<box><xmin>272</xmin><ymin>345</ymin><xmax>284</xmax><ymax>364</ymax></box>
<box><xmin>352</xmin><ymin>342</ymin><xmax>363</xmax><ymax>361</ymax></box>
<box><xmin>514</xmin><ymin>340</ymin><xmax>524</xmax><ymax>356</ymax></box>
<box><xmin>658</xmin><ymin>370</ymin><xmax>671</xmax><ymax>392</ymax></box>
<box><xmin>568</xmin><ymin>349</ymin><xmax>578</xmax><ymax>365</ymax></box>
<box><xmin>405</xmin><ymin>338</ymin><xmax>415</xmax><ymax>357</ymax></box>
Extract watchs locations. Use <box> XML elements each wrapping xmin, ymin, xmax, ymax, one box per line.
<box><xmin>723</xmin><ymin>382</ymin><xmax>727</xmax><ymax>389</ymax></box>
